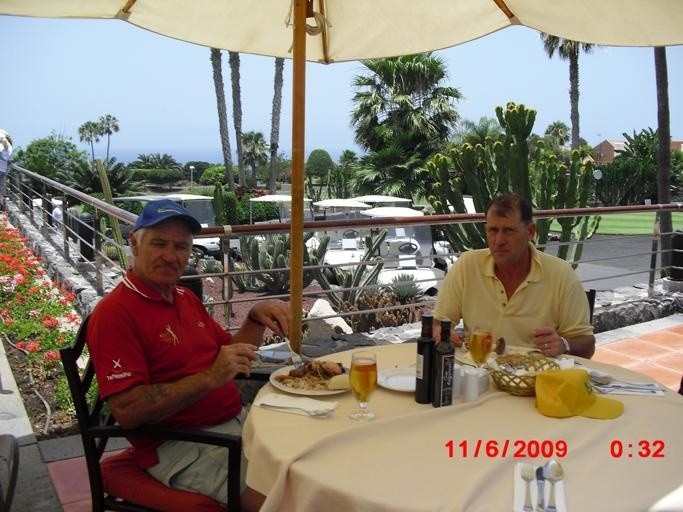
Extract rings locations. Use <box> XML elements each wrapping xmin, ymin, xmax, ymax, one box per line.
<box><xmin>544</xmin><ymin>344</ymin><xmax>547</xmax><ymax>349</ymax></box>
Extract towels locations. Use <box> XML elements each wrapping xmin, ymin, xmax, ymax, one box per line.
<box><xmin>510</xmin><ymin>461</ymin><xmax>565</xmax><ymax>512</ymax></box>
<box><xmin>251</xmin><ymin>391</ymin><xmax>340</xmax><ymax>420</ymax></box>
<box><xmin>586</xmin><ymin>376</ymin><xmax>665</xmax><ymax>398</ymax></box>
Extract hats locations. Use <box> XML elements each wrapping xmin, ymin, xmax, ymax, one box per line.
<box><xmin>131</xmin><ymin>199</ymin><xmax>201</xmax><ymax>233</ymax></box>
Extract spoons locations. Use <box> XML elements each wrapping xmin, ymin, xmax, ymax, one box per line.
<box><xmin>260</xmin><ymin>402</ymin><xmax>333</xmax><ymax>417</ymax></box>
<box><xmin>542</xmin><ymin>457</ymin><xmax>564</xmax><ymax>512</ymax></box>
<box><xmin>589</xmin><ymin>370</ymin><xmax>665</xmax><ymax>396</ymax></box>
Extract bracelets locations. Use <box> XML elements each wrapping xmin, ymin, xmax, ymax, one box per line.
<box><xmin>560</xmin><ymin>336</ymin><xmax>571</xmax><ymax>354</ymax></box>
<box><xmin>247</xmin><ymin>308</ymin><xmax>266</xmax><ymax>328</ymax></box>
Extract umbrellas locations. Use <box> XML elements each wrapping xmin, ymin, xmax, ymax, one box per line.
<box><xmin>0</xmin><ymin>0</ymin><xmax>683</xmax><ymax>356</ymax></box>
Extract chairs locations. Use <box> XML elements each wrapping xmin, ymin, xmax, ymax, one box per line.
<box><xmin>582</xmin><ymin>289</ymin><xmax>596</xmax><ymax>325</ymax></box>
<box><xmin>60</xmin><ymin>310</ymin><xmax>284</xmax><ymax>511</ymax></box>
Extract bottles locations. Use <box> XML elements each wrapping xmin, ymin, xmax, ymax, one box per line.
<box><xmin>413</xmin><ymin>310</ymin><xmax>482</xmax><ymax>409</ymax></box>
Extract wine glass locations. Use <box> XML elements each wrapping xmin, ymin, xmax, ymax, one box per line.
<box><xmin>468</xmin><ymin>325</ymin><xmax>494</xmax><ymax>369</ymax></box>
<box><xmin>347</xmin><ymin>349</ymin><xmax>378</xmax><ymax>423</ymax></box>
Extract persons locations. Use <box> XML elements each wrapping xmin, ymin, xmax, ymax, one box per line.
<box><xmin>87</xmin><ymin>200</ymin><xmax>292</xmax><ymax>512</ymax></box>
<box><xmin>426</xmin><ymin>192</ymin><xmax>596</xmax><ymax>359</ymax></box>
<box><xmin>0</xmin><ymin>129</ymin><xmax>13</xmax><ymax>210</ymax></box>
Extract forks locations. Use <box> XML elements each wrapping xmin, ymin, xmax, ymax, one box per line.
<box><xmin>519</xmin><ymin>460</ymin><xmax>535</xmax><ymax>512</ymax></box>
<box><xmin>275</xmin><ymin>324</ymin><xmax>304</xmax><ymax>370</ymax></box>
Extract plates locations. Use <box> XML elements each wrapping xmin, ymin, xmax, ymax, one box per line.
<box><xmin>453</xmin><ymin>350</ymin><xmax>519</xmax><ymax>369</ymax></box>
<box><xmin>376</xmin><ymin>366</ymin><xmax>418</xmax><ymax>393</ymax></box>
<box><xmin>268</xmin><ymin>362</ymin><xmax>354</xmax><ymax>397</ymax></box>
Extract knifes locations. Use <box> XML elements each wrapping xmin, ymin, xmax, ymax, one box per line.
<box><xmin>534</xmin><ymin>466</ymin><xmax>546</xmax><ymax>512</ymax></box>
<box><xmin>253</xmin><ymin>349</ymin><xmax>293</xmax><ymax>362</ymax></box>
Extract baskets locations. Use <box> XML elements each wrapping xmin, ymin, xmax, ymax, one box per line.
<box><xmin>485</xmin><ymin>352</ymin><xmax>560</xmax><ymax>398</ymax></box>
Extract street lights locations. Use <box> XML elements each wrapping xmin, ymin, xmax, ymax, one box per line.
<box><xmin>189</xmin><ymin>165</ymin><xmax>196</xmax><ymax>192</ymax></box>
<box><xmin>591</xmin><ymin>169</ymin><xmax>603</xmax><ymax>206</ymax></box>
